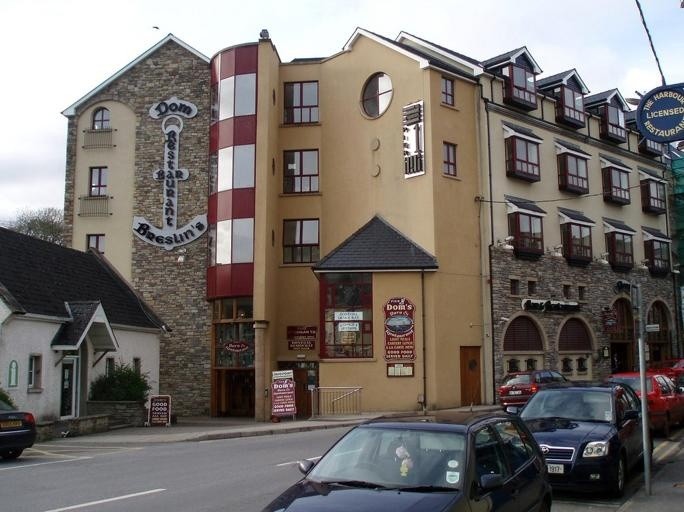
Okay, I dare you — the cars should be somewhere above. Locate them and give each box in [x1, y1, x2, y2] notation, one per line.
[0, 388, 35, 465]
[645, 358, 684, 387]
[518, 379, 655, 497]
[267, 412, 551, 510]
[497, 368, 567, 409]
[605, 370, 684, 436]
